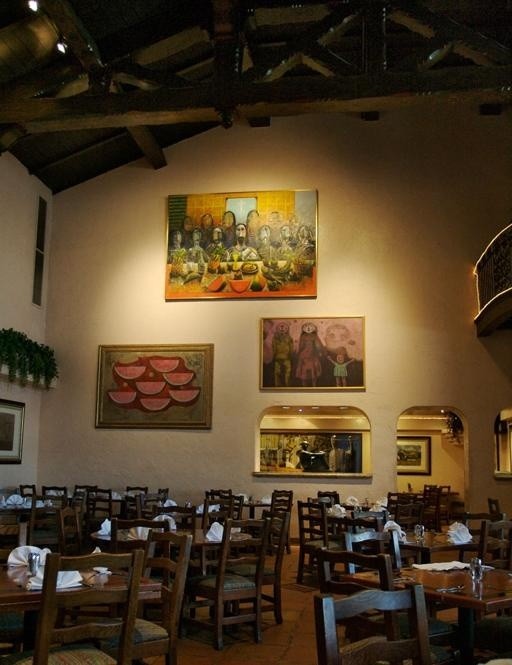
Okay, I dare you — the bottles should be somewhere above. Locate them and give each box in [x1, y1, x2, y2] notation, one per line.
[198, 253, 205, 275]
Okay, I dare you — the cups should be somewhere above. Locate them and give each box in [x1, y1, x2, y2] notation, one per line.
[297, 450, 325, 471]
[415, 525, 424, 540]
[470, 558, 484, 582]
[28, 552, 40, 575]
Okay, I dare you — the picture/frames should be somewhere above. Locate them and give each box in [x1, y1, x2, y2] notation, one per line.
[398, 434, 430, 475]
[166, 189, 319, 300]
[1, 399, 24, 464]
[259, 317, 366, 391]
[261, 429, 371, 472]
[97, 345, 213, 430]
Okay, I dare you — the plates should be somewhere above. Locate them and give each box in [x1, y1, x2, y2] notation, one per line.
[186, 256, 195, 273]
[241, 263, 258, 274]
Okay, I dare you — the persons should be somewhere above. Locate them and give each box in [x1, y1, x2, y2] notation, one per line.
[328, 433, 355, 473]
[295, 441, 328, 472]
